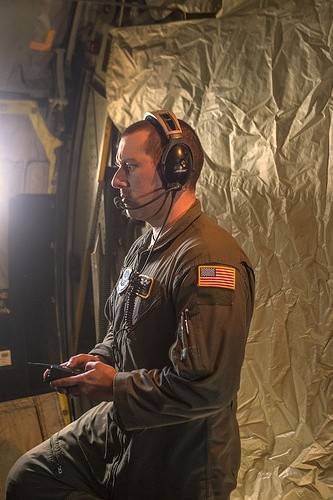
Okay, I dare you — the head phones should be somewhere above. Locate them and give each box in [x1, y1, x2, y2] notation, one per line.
[142, 109, 196, 191]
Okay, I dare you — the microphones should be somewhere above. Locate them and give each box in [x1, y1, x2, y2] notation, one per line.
[113, 189, 173, 211]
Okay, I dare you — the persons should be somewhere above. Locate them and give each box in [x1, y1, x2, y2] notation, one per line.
[2, 110, 256, 500]
